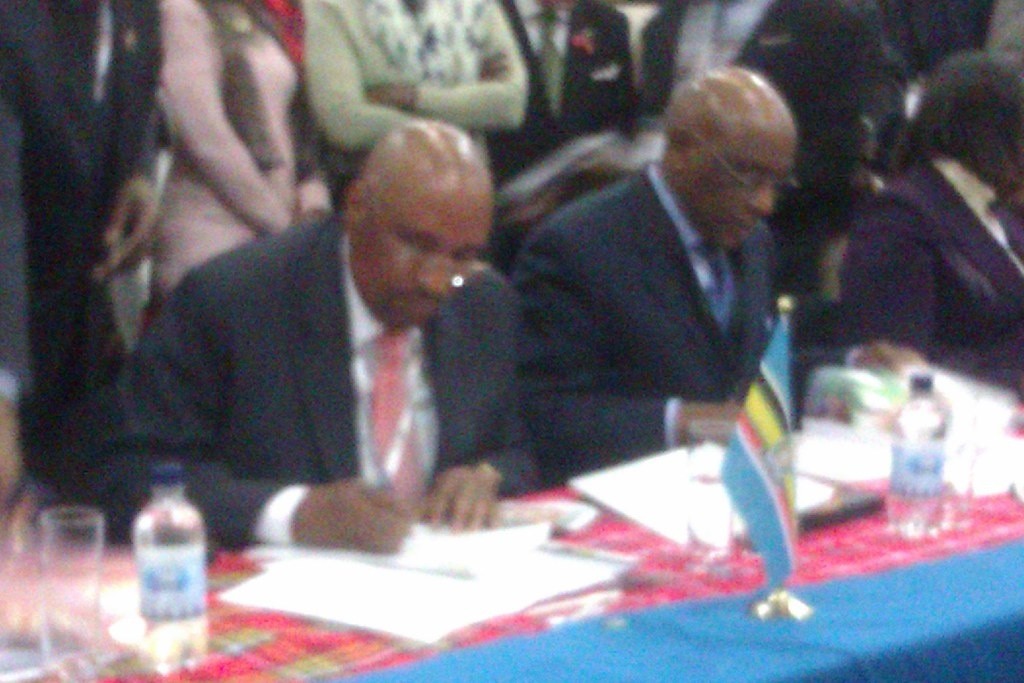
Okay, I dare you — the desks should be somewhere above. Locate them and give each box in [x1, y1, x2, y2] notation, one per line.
[0, 401, 1024, 683]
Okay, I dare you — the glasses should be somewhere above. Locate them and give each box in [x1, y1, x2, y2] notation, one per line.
[691, 133, 799, 203]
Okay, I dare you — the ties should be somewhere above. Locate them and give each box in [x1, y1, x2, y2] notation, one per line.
[695, 241, 736, 341]
[533, 8, 566, 120]
[991, 200, 1024, 266]
[365, 329, 427, 512]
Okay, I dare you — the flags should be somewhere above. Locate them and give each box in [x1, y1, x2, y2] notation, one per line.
[717, 314, 797, 589]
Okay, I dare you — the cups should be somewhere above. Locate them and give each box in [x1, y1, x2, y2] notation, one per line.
[36, 506, 106, 670]
[686, 421, 740, 563]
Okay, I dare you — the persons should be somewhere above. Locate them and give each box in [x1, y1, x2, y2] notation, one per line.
[838, 50, 1024, 406]
[509, 67, 926, 493]
[0, 0, 1023, 505]
[59, 120, 566, 553]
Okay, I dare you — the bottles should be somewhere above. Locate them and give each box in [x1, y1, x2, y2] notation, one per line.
[888, 374, 950, 542]
[128, 462, 210, 678]
